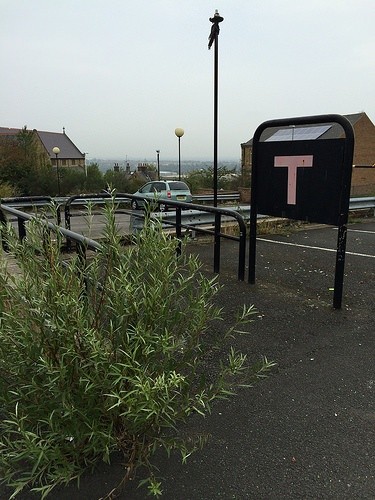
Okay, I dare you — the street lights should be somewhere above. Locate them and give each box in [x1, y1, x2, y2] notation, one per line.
[175, 128, 185, 180]
[52, 147, 60, 196]
[156, 149, 160, 180]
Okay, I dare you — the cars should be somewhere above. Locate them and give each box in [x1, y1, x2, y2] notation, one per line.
[130, 179, 192, 212]
